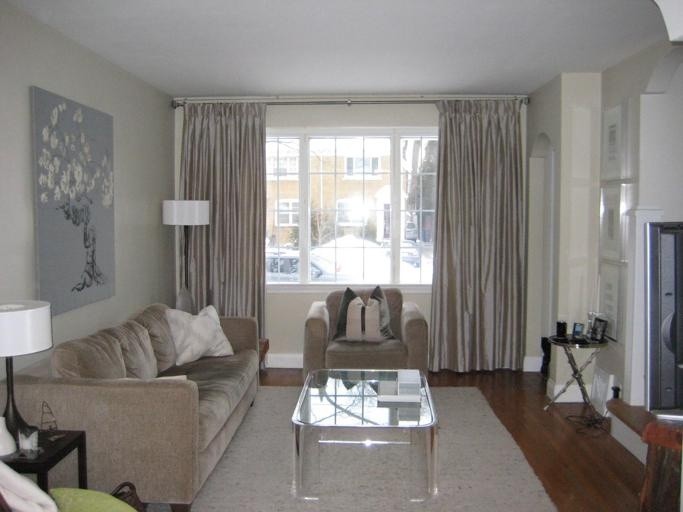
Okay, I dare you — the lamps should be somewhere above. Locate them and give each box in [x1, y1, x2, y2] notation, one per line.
[160, 201, 213, 290]
[0, 299, 52, 455]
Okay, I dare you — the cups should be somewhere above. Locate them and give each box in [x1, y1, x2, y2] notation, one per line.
[556, 322, 566, 339]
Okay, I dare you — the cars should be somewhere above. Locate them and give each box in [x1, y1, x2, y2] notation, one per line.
[380, 221, 420, 267]
[265, 247, 350, 282]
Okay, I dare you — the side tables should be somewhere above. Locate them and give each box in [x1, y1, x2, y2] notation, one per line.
[6, 429, 87, 501]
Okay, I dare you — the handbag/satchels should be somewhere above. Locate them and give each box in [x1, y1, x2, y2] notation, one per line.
[111, 482, 145, 512]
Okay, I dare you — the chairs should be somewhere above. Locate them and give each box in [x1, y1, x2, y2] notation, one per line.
[301, 286, 429, 391]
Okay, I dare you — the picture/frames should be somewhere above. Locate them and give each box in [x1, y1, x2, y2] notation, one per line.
[30, 85, 115, 317]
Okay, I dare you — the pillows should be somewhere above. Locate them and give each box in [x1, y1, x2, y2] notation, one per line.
[331, 283, 395, 344]
[47, 299, 234, 388]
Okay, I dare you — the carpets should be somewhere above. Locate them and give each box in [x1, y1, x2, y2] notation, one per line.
[139, 385, 558, 511]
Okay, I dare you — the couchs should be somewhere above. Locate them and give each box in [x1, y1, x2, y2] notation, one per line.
[0, 315, 263, 511]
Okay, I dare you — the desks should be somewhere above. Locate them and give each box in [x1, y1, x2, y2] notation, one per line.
[543, 334, 609, 414]
